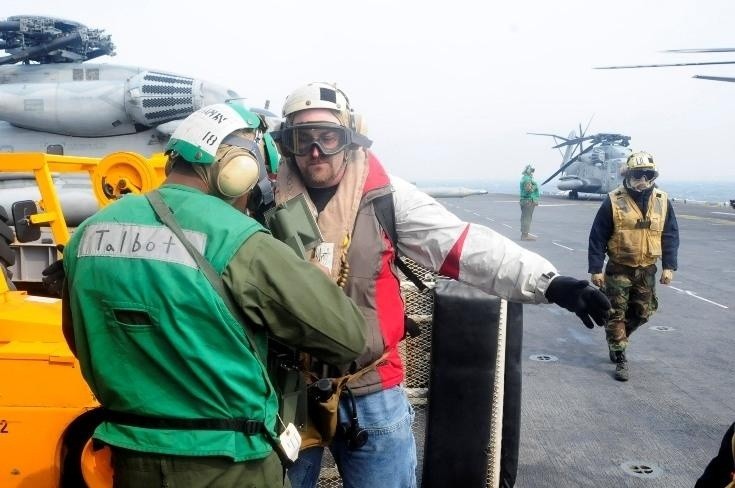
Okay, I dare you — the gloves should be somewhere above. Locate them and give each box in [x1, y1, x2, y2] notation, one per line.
[660, 270, 673, 284]
[545, 274, 610, 328]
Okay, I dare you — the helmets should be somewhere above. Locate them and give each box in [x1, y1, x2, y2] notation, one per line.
[282, 83, 355, 130]
[165, 102, 281, 199]
[621, 152, 657, 174]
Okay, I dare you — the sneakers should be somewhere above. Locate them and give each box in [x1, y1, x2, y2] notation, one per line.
[610, 349, 631, 381]
[521, 233, 538, 241]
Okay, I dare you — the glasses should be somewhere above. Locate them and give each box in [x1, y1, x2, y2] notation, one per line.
[280, 121, 353, 156]
[630, 170, 655, 179]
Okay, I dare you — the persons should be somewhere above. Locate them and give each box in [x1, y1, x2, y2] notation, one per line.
[518, 164, 541, 241]
[587, 150, 680, 383]
[38, 80, 611, 487]
[58, 101, 369, 487]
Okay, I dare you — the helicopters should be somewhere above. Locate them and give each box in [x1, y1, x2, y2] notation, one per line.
[595, 42, 735, 91]
[0, 6, 489, 289]
[526, 111, 635, 196]
